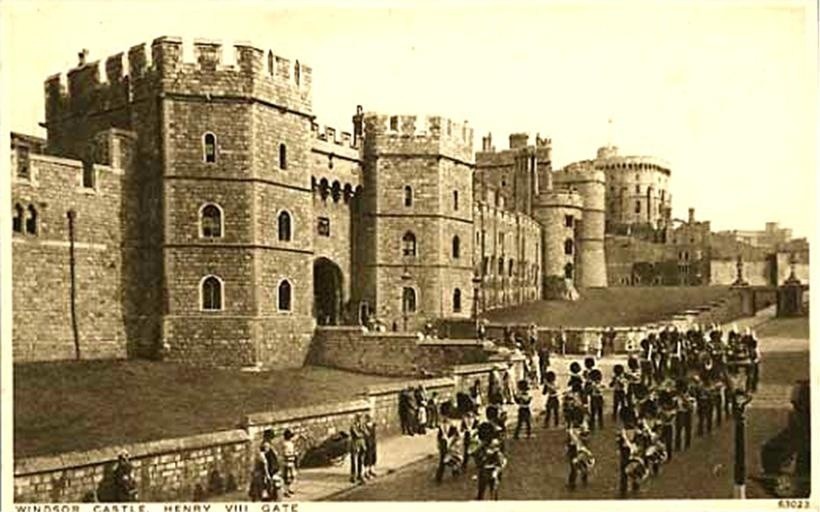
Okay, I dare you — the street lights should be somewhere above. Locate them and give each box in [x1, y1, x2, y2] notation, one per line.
[472, 269, 481, 340]
[722, 358, 752, 499]
[402, 266, 412, 332]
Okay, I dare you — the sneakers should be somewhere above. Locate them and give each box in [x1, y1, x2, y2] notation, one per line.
[348, 468, 378, 487]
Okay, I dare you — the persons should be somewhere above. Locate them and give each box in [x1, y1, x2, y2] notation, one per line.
[248, 440, 275, 501]
[351, 104, 365, 145]
[347, 411, 368, 485]
[359, 413, 377, 481]
[116, 449, 140, 501]
[278, 427, 299, 498]
[395, 311, 764, 498]
[259, 429, 280, 501]
